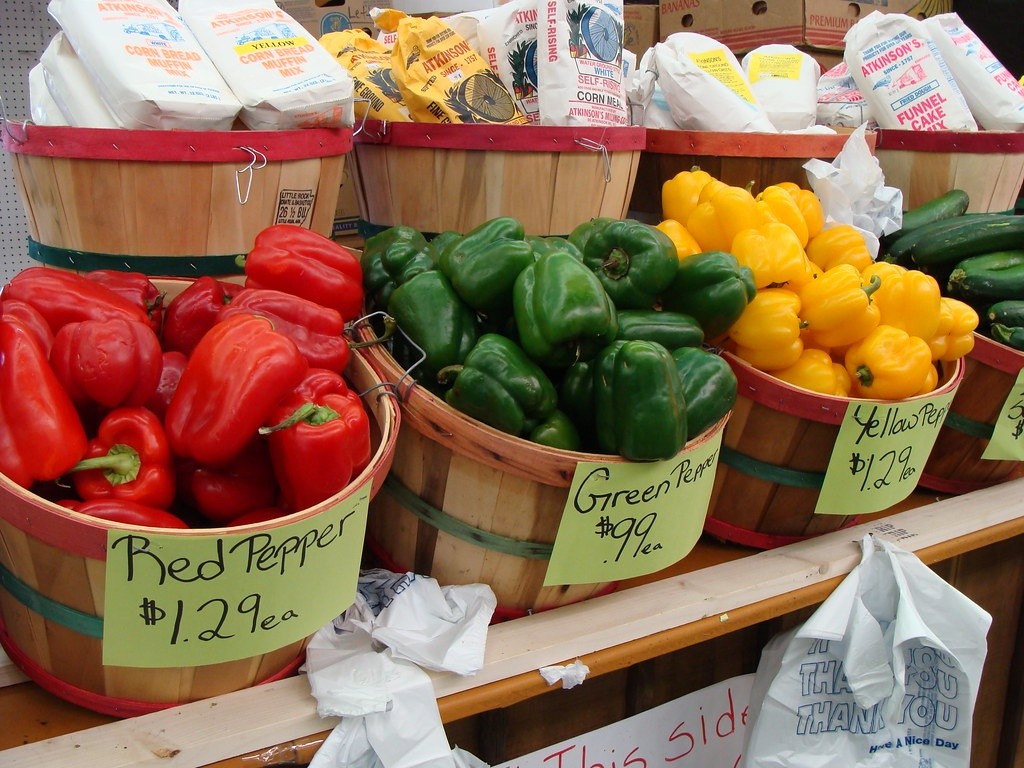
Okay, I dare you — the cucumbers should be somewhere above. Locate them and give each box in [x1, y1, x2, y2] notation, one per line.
[878, 190, 1024, 356]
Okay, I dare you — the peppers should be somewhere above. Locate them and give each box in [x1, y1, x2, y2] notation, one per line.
[0, 170, 979, 528]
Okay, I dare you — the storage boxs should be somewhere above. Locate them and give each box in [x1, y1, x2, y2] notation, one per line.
[278, 0, 962, 250]
